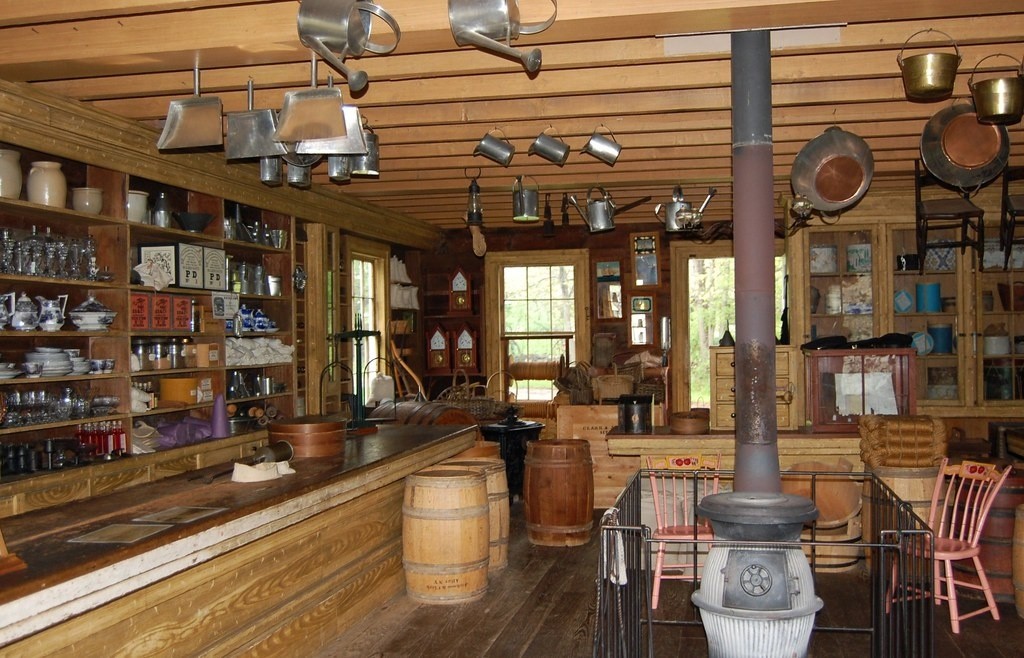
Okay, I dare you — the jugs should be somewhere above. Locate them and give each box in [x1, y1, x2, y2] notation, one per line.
[237, 260, 265, 295]
[448, 0, 557, 73]
[0, 291, 69, 331]
[296, 0, 402, 91]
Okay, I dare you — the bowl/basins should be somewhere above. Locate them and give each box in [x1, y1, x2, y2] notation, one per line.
[172, 212, 218, 232]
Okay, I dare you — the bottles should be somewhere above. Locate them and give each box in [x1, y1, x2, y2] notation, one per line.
[0, 223, 110, 280]
[152, 192, 171, 228]
[132, 380, 157, 413]
[77, 420, 127, 461]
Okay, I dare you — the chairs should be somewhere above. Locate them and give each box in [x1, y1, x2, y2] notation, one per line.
[915, 158, 985, 275]
[882, 457, 1012, 634]
[644, 450, 723, 610]
[997, 159, 1024, 272]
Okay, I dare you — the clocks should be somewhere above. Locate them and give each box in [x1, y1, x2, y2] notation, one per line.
[423, 265, 479, 375]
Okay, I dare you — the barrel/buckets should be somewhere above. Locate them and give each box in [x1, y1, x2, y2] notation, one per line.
[897, 28, 1024, 124]
[524, 439, 594, 546]
[370, 401, 510, 605]
[862, 464, 1024, 619]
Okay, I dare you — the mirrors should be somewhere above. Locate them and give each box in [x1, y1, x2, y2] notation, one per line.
[591, 230, 661, 351]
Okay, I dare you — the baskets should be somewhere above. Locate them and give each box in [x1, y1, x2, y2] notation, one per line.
[552, 352, 667, 405]
[432, 369, 524, 421]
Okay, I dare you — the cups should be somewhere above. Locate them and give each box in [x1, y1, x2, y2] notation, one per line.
[0, 390, 53, 430]
[471, 126, 622, 170]
[88, 358, 115, 375]
[22, 361, 43, 378]
[132, 387, 149, 413]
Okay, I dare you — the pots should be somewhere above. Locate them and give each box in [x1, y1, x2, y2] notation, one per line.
[790, 127, 875, 220]
[922, 97, 1010, 195]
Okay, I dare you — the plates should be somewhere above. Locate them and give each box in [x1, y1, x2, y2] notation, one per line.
[0, 346, 90, 380]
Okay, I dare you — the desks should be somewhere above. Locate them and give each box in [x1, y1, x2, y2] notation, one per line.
[606, 425, 863, 486]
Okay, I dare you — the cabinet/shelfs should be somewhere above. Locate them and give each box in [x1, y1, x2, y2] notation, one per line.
[295, 217, 353, 419]
[0, 139, 295, 520]
[783, 191, 1024, 435]
[707, 343, 801, 433]
